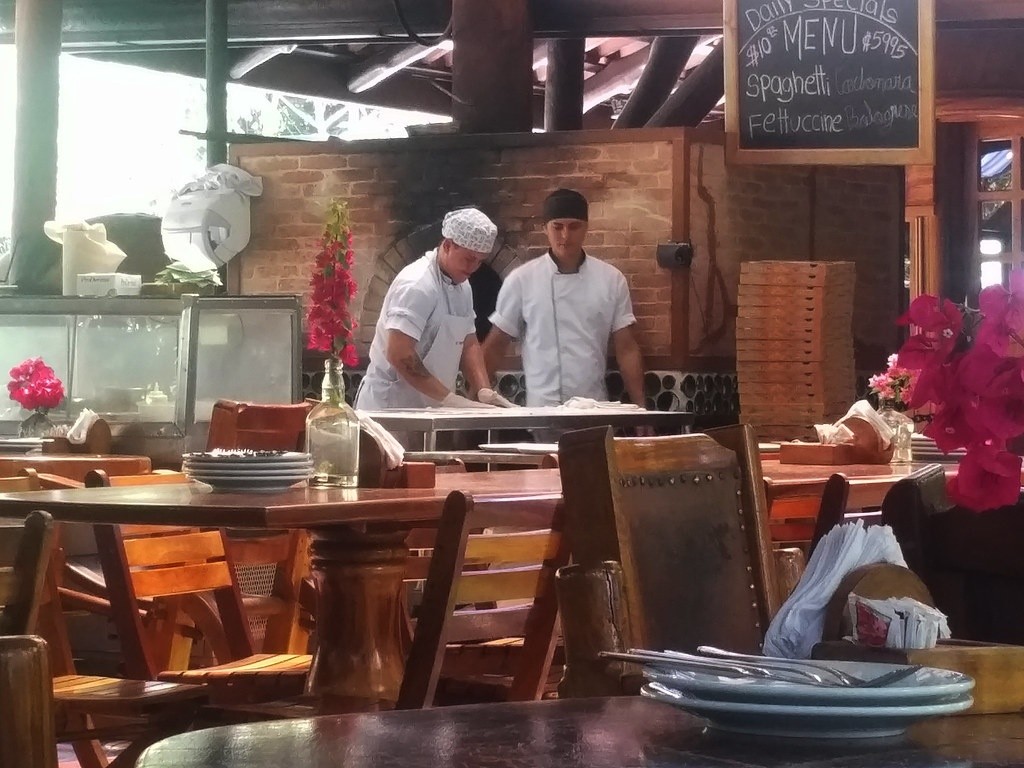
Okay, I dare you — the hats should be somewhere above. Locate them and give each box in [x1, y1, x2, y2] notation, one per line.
[542, 188, 588, 224]
[442, 207, 498, 253]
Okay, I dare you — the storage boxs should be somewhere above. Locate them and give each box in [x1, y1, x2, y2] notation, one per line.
[736, 259, 858, 442]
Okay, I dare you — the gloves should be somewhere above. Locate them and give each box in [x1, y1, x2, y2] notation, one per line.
[441, 391, 497, 408]
[478, 388, 521, 408]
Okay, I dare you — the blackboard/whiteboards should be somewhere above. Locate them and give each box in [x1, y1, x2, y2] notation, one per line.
[722, 0, 931, 163]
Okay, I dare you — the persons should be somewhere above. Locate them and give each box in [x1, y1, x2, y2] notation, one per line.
[352, 208, 520, 452]
[468, 188, 654, 443]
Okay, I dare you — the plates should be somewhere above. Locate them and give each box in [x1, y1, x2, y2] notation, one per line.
[911, 432, 968, 457]
[640, 661, 976, 737]
[183, 450, 315, 493]
[0, 437, 54, 451]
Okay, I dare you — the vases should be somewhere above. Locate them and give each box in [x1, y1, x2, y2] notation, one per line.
[876, 399, 915, 463]
[305, 359, 360, 490]
[19, 411, 56, 439]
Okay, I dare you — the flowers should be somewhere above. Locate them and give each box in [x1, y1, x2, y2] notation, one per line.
[894, 268, 1024, 513]
[307, 194, 360, 368]
[6, 358, 64, 416]
[868, 353, 921, 412]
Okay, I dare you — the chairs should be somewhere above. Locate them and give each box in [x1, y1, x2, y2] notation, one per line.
[0, 395, 1024, 768]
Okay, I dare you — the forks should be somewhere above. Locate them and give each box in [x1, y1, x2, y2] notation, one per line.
[599, 644, 922, 687]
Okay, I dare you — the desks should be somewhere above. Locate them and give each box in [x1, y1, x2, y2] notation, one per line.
[0, 463, 1024, 704]
[353, 406, 694, 471]
[135, 695, 1024, 768]
[0, 453, 152, 483]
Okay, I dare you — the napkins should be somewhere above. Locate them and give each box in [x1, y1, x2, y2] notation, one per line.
[762, 518, 908, 660]
[833, 400, 897, 451]
[354, 409, 405, 469]
[66, 408, 100, 445]
